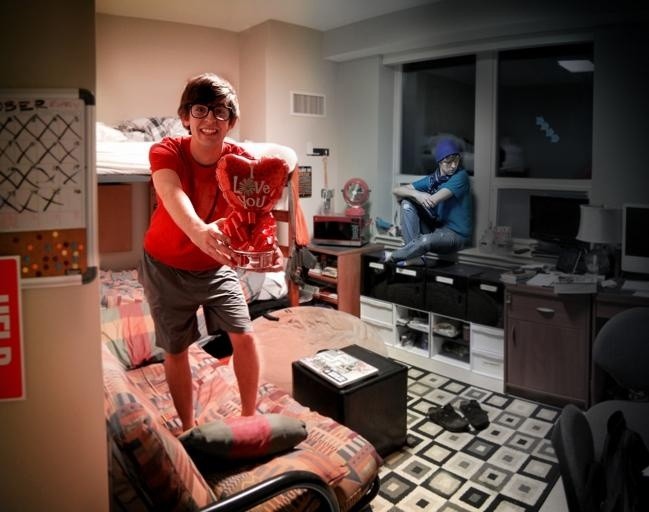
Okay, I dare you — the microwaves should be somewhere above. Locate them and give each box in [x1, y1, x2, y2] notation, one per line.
[312, 212, 371, 248]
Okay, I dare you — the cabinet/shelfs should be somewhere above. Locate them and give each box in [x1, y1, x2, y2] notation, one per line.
[355, 246, 504, 395]
[501, 275, 594, 414]
[295, 240, 387, 317]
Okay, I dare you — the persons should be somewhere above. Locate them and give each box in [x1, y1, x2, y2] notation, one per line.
[139, 69, 259, 432]
[375, 141, 473, 274]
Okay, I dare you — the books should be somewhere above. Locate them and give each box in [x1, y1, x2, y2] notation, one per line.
[499, 270, 598, 295]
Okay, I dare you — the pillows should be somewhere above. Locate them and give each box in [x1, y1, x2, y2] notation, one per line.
[174, 411, 307, 468]
[97, 297, 168, 366]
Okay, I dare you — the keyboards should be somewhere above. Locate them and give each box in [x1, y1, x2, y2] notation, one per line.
[620, 278, 649, 292]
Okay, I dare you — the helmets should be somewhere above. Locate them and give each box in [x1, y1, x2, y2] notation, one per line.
[433, 139, 462, 162]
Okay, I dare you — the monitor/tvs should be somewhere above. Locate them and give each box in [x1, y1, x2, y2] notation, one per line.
[528, 194, 588, 242]
[622, 203, 649, 273]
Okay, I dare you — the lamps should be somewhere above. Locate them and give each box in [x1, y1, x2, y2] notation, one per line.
[573, 203, 610, 275]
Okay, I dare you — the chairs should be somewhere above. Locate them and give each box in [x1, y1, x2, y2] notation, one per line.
[579, 305, 648, 465]
[548, 403, 648, 511]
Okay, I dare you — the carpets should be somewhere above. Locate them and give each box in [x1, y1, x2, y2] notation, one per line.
[370, 353, 574, 512]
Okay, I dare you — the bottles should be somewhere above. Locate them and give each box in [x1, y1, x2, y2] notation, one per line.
[400, 336, 407, 346]
[479, 222, 495, 254]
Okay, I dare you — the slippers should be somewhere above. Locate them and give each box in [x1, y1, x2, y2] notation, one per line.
[429, 398, 491, 432]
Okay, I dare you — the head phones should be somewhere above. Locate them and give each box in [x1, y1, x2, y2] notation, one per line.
[430, 169, 451, 186]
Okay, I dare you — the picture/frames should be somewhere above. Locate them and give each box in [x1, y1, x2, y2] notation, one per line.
[1, 86, 99, 293]
[557, 244, 584, 274]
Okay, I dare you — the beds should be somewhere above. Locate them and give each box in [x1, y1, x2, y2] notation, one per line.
[96, 121, 304, 372]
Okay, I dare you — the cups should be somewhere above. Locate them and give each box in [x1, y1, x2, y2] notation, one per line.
[463, 326, 470, 343]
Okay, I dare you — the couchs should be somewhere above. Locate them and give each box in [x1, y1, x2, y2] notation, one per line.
[98, 331, 385, 510]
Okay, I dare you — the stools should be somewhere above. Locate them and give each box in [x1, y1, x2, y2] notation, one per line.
[229, 303, 389, 396]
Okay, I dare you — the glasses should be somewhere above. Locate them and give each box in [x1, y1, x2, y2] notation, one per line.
[187, 101, 232, 123]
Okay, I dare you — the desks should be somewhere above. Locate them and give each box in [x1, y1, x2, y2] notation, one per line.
[591, 283, 649, 413]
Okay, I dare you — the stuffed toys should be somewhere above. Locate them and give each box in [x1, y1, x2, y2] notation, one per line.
[215, 154, 289, 266]
